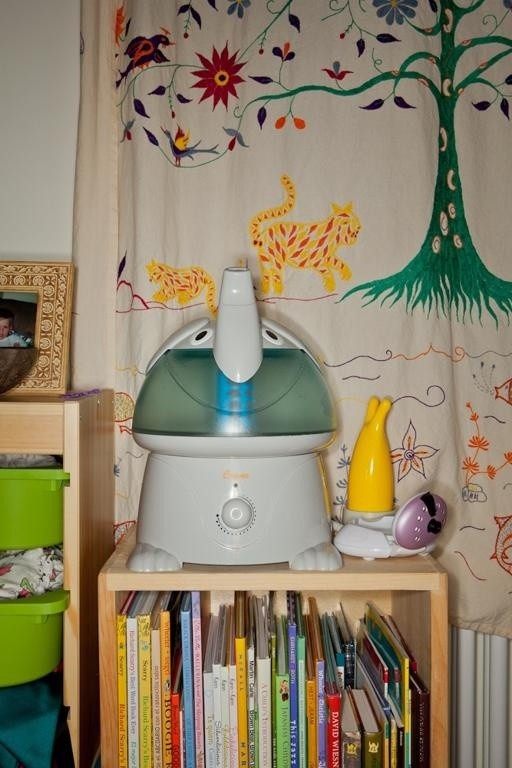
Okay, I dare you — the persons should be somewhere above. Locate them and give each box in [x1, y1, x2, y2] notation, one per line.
[0, 307, 28, 347]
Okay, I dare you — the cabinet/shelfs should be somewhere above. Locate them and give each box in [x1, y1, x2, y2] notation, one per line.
[0, 387, 116, 767]
[96, 572, 449, 768]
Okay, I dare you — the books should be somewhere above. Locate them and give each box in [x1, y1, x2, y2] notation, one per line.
[113, 589, 434, 768]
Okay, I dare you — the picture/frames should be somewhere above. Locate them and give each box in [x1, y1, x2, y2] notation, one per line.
[0, 260, 74, 397]
